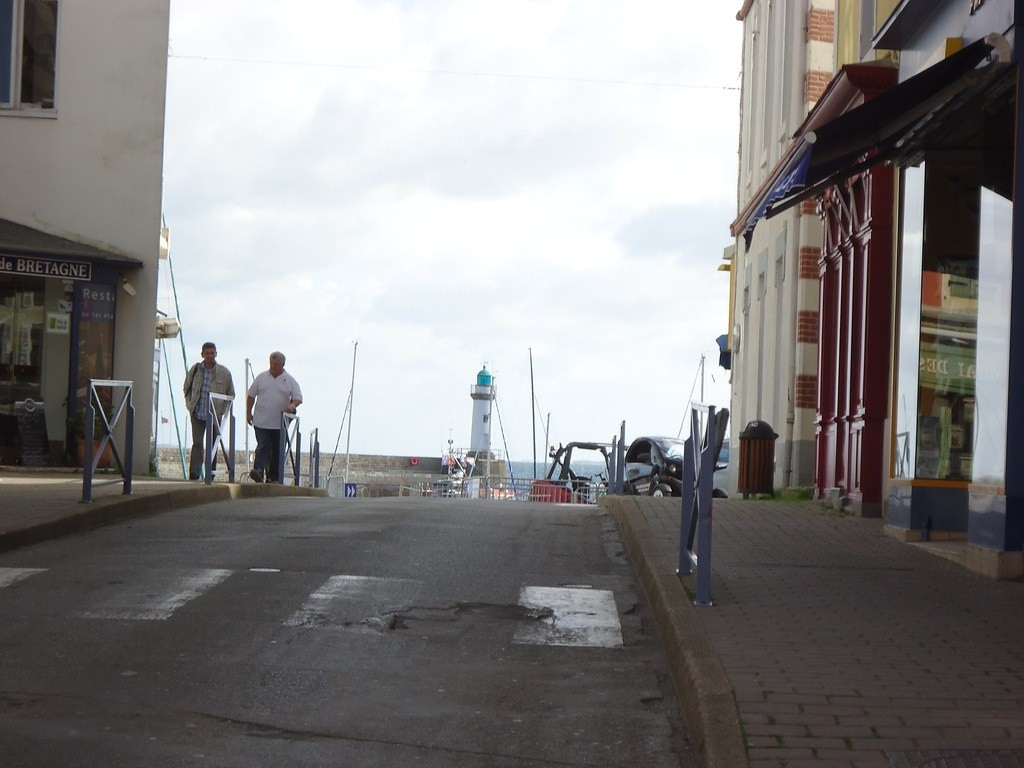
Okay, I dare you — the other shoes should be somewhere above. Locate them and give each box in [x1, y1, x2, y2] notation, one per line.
[190, 472, 199, 480]
[250, 467, 263, 483]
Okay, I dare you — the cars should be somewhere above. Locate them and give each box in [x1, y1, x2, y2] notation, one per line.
[713, 438, 729, 499]
[623, 436, 686, 497]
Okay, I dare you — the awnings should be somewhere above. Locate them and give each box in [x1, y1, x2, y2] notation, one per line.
[746, 32, 1011, 232]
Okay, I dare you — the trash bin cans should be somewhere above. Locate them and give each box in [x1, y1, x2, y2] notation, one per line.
[736, 422, 779, 500]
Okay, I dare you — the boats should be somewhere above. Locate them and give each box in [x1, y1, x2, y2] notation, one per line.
[428, 347, 629, 502]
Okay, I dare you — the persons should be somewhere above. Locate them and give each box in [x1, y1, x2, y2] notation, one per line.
[183, 343, 235, 478]
[245, 352, 302, 483]
